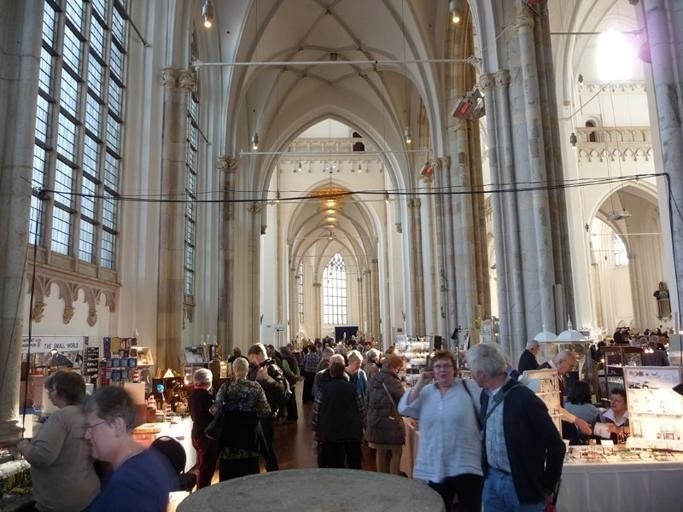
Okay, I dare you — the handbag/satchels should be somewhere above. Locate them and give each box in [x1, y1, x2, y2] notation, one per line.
[363, 410, 406, 445]
[203, 380, 233, 442]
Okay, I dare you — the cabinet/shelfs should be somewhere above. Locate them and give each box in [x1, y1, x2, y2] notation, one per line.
[599, 346, 664, 401]
[84, 347, 99, 375]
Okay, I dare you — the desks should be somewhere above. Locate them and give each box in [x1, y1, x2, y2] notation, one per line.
[555, 444, 683, 512]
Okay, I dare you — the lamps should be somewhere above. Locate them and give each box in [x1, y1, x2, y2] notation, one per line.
[600, 86, 633, 221]
[534, 323, 556, 343]
[552, 315, 590, 345]
[578, 74, 583, 85]
[451, 86, 482, 120]
[250, 0, 259, 150]
[201, 0, 214, 28]
[570, 133, 577, 148]
[403, 0, 413, 144]
[449, 0, 461, 23]
[421, 155, 440, 178]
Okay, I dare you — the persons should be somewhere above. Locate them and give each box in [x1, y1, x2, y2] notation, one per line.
[466, 342, 566, 512]
[397, 351, 486, 512]
[518, 340, 630, 446]
[15, 369, 180, 512]
[188, 336, 405, 491]
[641, 335, 669, 366]
